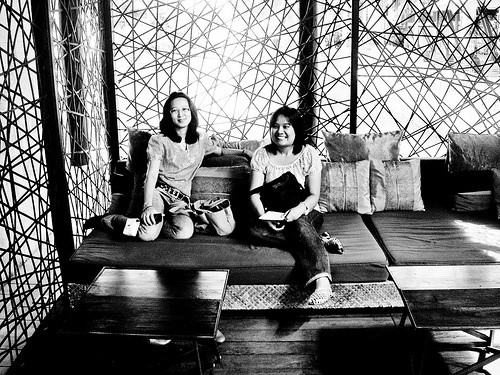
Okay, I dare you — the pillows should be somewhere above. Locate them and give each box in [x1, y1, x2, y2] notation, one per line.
[125, 128, 500, 222]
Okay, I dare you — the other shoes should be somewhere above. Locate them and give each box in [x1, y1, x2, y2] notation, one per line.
[321, 231, 344, 254]
[307, 289, 335, 304]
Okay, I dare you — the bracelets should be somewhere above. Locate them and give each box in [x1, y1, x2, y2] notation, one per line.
[300, 202, 309, 215]
[242, 149, 245, 154]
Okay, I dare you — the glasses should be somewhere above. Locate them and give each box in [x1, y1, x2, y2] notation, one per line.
[169, 107, 190, 114]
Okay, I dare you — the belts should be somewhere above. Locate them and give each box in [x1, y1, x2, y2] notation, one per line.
[158, 182, 189, 202]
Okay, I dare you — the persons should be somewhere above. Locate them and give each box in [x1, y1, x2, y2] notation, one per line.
[249, 106, 332, 305]
[83, 91, 251, 242]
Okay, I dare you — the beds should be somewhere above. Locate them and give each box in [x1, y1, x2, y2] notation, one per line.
[362, 160, 500, 314]
[67, 161, 389, 319]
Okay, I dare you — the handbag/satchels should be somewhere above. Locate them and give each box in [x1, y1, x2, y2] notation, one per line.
[249, 170, 307, 211]
[194, 196, 235, 235]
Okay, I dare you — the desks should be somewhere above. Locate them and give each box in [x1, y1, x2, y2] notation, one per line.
[384, 262, 500, 375]
[56, 265, 230, 375]
